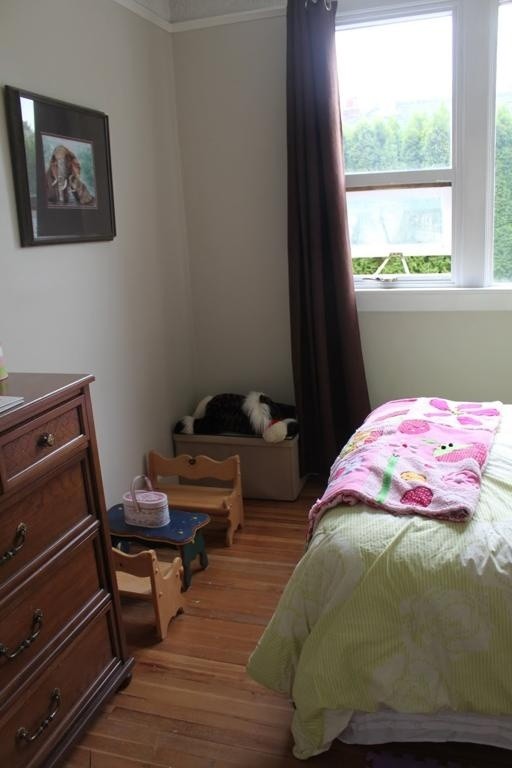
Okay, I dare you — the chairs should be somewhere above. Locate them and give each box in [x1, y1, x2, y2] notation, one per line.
[143, 448, 246, 548]
[111, 548, 188, 642]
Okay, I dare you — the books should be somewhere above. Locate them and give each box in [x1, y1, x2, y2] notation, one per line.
[1, 395, 24, 416]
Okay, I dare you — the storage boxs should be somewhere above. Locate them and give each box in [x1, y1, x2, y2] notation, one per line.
[172, 432, 311, 502]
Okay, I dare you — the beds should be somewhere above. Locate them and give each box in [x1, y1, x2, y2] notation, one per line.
[246, 394, 512, 760]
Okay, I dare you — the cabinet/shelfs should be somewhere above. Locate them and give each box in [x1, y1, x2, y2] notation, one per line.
[0, 371, 137, 767]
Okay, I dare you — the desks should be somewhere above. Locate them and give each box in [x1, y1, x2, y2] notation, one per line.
[107, 501, 210, 594]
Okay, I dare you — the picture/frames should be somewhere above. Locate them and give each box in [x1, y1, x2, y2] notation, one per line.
[2, 83, 117, 248]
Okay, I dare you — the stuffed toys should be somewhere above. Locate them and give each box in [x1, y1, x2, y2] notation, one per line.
[174, 391, 298, 444]
